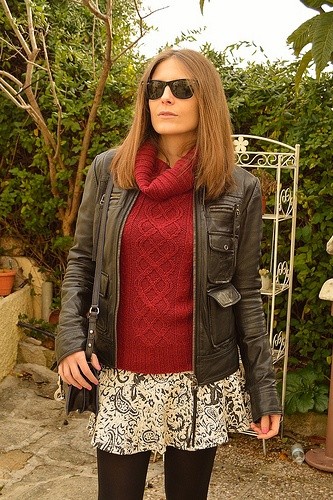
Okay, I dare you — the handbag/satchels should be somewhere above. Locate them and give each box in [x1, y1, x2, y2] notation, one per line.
[66, 358, 100, 418]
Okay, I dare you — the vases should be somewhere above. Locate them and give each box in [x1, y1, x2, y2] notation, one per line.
[0, 269, 16, 296]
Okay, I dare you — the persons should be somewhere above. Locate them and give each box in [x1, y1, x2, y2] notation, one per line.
[56, 49, 283, 500]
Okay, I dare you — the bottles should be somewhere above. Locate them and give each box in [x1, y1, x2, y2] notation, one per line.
[292, 444, 305, 466]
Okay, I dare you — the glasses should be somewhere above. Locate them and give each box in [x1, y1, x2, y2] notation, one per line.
[146, 79, 198, 100]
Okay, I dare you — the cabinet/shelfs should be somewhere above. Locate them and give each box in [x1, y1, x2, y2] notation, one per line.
[232, 134, 300, 455]
